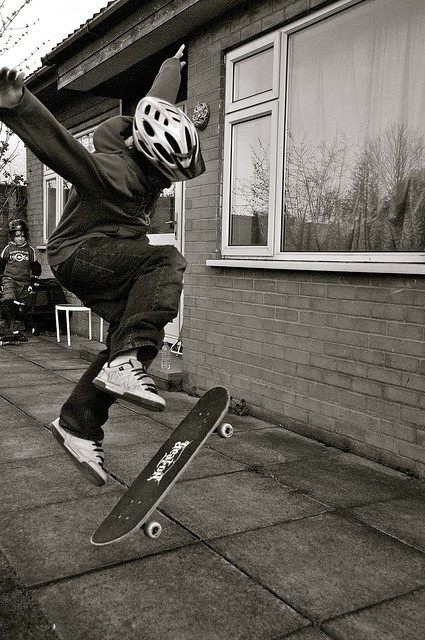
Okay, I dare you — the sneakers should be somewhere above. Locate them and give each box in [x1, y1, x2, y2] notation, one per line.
[48, 416, 108, 487]
[91, 357, 167, 413]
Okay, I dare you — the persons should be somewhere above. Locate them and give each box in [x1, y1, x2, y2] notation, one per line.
[1, 218, 42, 342]
[0, 44, 206, 491]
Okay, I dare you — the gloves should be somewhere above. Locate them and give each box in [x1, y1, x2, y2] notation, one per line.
[29, 278, 38, 291]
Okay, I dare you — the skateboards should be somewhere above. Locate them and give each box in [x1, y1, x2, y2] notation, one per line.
[91, 386, 233, 546]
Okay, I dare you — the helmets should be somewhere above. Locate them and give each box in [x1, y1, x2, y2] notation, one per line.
[132, 96, 206, 183]
[6, 219, 29, 232]
